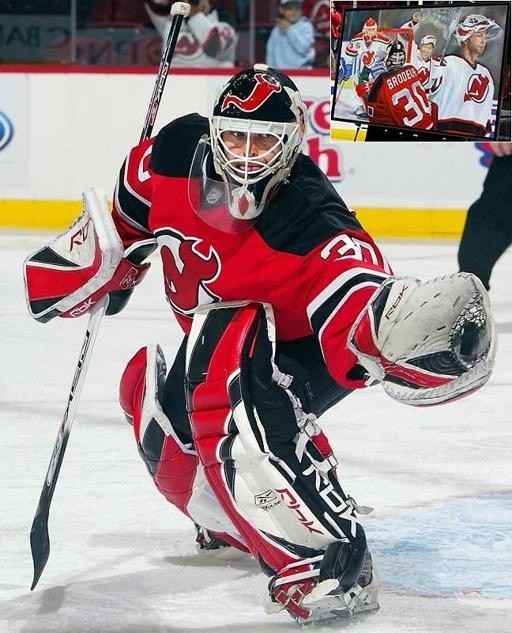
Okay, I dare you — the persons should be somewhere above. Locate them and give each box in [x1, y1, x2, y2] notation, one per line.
[22, 63, 495, 612]
[265, 0, 317, 69]
[331, 2, 504, 136]
[144, 1, 238, 68]
[460, 144, 512, 293]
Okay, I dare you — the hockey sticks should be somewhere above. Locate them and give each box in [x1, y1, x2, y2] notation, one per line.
[30, 2, 191, 593]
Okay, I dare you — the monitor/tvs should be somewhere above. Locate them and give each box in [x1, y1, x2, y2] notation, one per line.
[331, 1, 511, 141]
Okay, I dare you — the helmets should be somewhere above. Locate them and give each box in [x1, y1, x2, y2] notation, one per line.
[207, 64, 309, 222]
[384, 41, 408, 71]
[364, 19, 379, 35]
[422, 34, 438, 46]
[454, 15, 503, 45]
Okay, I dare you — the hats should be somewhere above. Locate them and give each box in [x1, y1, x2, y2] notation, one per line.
[278, 0, 303, 9]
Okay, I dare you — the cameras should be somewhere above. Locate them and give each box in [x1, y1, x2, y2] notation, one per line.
[188, 0, 200, 6]
